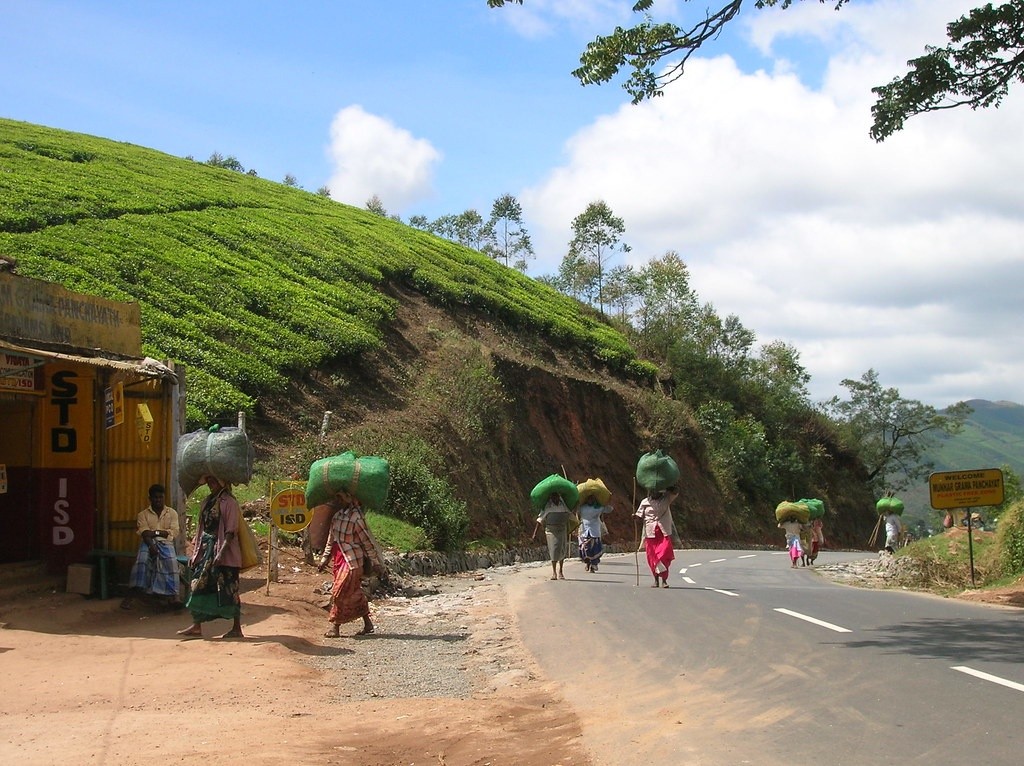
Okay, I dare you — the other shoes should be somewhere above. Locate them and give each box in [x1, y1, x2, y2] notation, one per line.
[120, 597, 140, 609]
[651, 581, 669, 588]
[175, 628, 204, 638]
[323, 629, 340, 638]
[355, 626, 374, 635]
[222, 628, 244, 638]
[585, 564, 590, 571]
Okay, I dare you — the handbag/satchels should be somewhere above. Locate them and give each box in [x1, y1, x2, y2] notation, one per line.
[800, 540, 806, 544]
[817, 531, 823, 544]
[363, 553, 373, 575]
[567, 512, 580, 535]
[235, 503, 263, 573]
[600, 520, 609, 535]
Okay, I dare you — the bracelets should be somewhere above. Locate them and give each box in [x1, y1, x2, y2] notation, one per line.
[155, 531, 160, 535]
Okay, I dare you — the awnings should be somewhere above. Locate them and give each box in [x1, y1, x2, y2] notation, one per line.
[0, 334, 172, 391]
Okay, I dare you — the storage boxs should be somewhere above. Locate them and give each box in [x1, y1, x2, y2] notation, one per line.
[65, 564, 96, 596]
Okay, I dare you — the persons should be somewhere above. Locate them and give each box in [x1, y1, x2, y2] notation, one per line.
[879, 510, 902, 552]
[120, 485, 179, 610]
[778, 515, 826, 568]
[318, 488, 381, 636]
[576, 495, 613, 573]
[631, 486, 680, 587]
[532, 492, 581, 580]
[175, 476, 242, 637]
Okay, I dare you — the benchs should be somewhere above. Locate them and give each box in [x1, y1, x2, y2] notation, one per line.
[87, 548, 190, 604]
